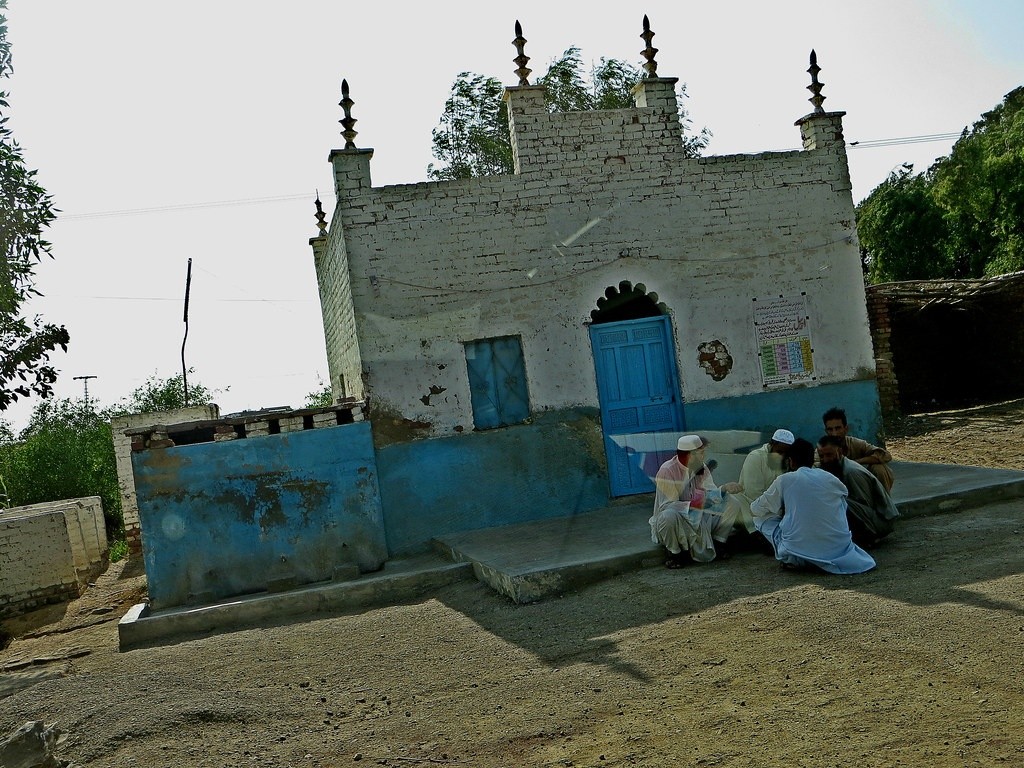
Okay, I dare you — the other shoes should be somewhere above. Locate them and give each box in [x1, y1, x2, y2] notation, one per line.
[665, 554, 681, 570]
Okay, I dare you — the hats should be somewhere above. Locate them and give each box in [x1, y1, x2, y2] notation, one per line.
[771, 429, 795, 445]
[677, 435, 703, 451]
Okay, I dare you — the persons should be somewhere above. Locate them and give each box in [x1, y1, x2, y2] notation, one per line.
[730, 429, 795, 557]
[653, 434, 741, 570]
[750, 437, 877, 575]
[814, 406, 894, 496]
[814, 435, 902, 550]
